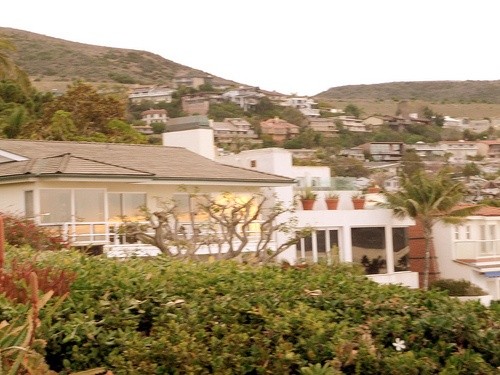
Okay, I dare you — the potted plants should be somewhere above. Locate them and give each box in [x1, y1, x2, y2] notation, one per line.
[324, 192, 339, 210]
[351, 190, 367, 211]
[297, 184, 318, 211]
